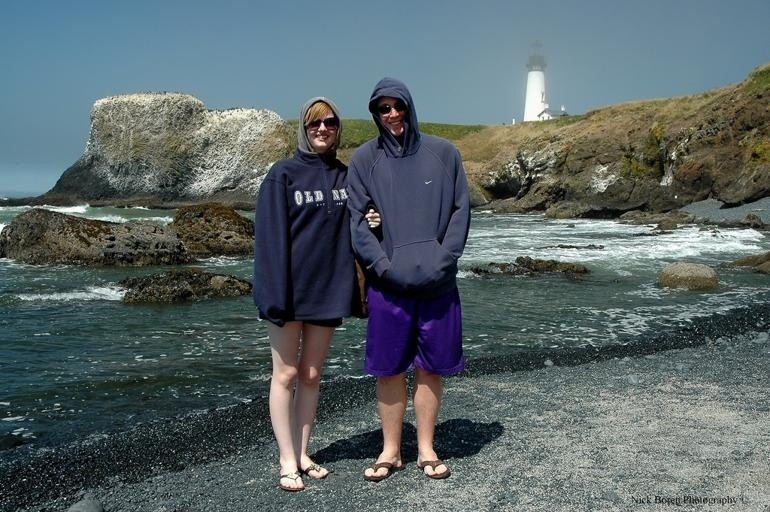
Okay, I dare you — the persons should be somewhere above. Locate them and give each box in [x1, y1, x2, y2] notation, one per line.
[252, 96, 380, 491]
[347, 77, 471, 481]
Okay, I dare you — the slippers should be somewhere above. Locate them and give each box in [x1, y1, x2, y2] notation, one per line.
[416, 456, 451, 479]
[363, 458, 406, 481]
[301, 461, 330, 480]
[279, 469, 305, 492]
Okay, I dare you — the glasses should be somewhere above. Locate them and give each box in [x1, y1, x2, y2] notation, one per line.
[376, 101, 406, 114]
[308, 118, 339, 131]
